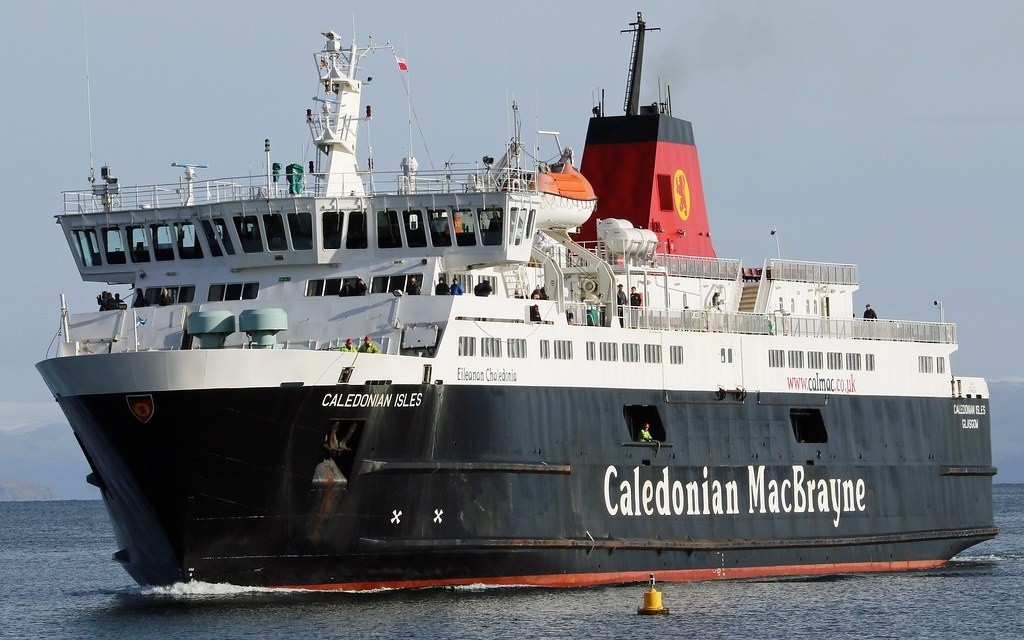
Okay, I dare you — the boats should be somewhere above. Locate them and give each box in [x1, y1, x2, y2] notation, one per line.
[495, 164, 597, 230]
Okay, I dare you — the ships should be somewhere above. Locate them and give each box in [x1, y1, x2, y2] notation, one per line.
[33, 11, 999, 591]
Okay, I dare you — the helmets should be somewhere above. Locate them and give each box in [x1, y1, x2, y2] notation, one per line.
[364, 336, 371, 340]
[346, 339, 352, 344]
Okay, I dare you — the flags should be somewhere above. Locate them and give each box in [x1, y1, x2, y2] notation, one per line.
[136, 316, 147, 326]
[396, 58, 408, 71]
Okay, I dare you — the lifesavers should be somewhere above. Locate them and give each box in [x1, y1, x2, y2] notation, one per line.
[583, 280, 596, 292]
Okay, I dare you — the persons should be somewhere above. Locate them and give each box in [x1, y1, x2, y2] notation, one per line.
[638, 423, 652, 442]
[435, 277, 463, 295]
[96, 291, 124, 312]
[864, 305, 878, 322]
[432, 212, 448, 246]
[617, 284, 627, 328]
[357, 336, 378, 353]
[521, 284, 546, 321]
[158, 288, 173, 305]
[133, 288, 145, 307]
[631, 286, 642, 309]
[475, 280, 492, 321]
[489, 212, 500, 229]
[339, 278, 367, 296]
[408, 278, 420, 295]
[340, 339, 357, 352]
